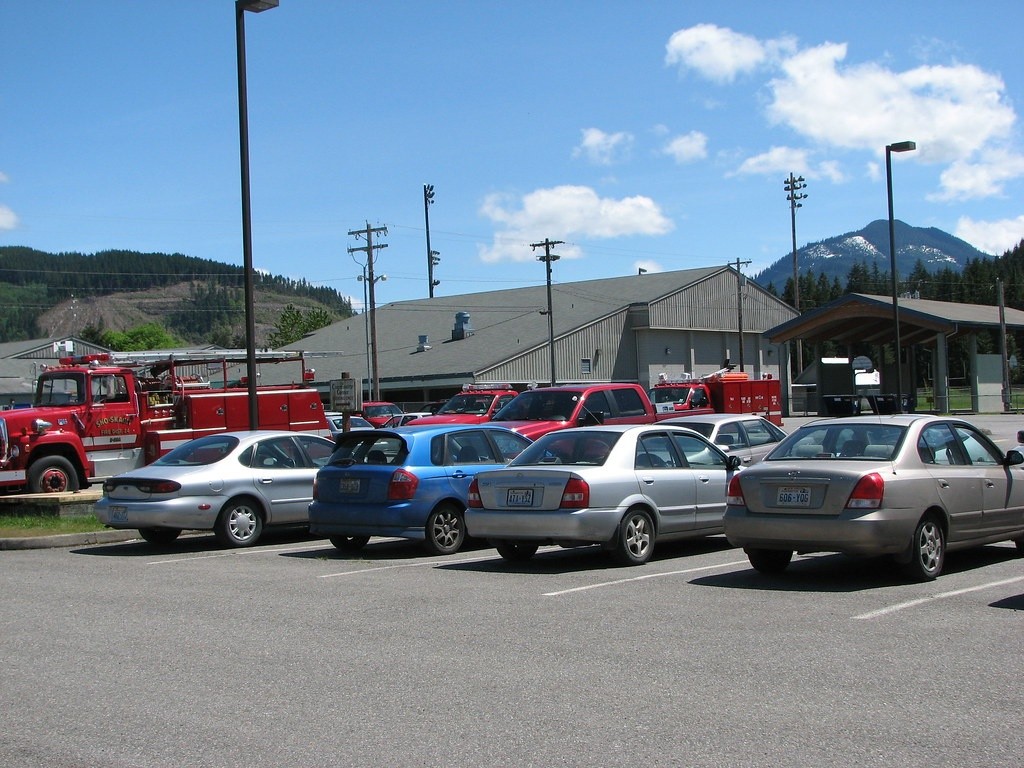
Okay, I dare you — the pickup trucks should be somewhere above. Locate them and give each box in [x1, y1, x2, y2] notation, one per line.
[480, 382, 715, 462]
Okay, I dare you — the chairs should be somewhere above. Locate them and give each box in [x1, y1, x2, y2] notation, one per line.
[466, 399, 508, 411]
[367, 450, 387, 464]
[801, 439, 938, 463]
[459, 444, 482, 462]
[639, 451, 668, 469]
[559, 399, 585, 419]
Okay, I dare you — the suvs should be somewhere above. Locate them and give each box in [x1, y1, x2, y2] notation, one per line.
[353, 402, 408, 430]
[405, 383, 520, 426]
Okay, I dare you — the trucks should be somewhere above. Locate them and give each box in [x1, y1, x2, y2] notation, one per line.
[645, 372, 784, 428]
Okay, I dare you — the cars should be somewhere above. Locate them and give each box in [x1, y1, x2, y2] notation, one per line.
[463, 424, 750, 568]
[637, 413, 816, 468]
[379, 412, 432, 429]
[324, 412, 389, 456]
[307, 424, 559, 555]
[93, 429, 369, 549]
[723, 414, 1024, 584]
[894, 411, 993, 463]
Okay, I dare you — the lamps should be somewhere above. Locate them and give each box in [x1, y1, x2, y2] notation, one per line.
[667, 348, 670, 354]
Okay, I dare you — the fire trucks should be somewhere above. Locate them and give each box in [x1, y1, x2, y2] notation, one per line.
[0, 347, 333, 512]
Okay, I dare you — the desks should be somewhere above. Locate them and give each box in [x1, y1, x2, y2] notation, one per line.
[822, 394, 904, 420]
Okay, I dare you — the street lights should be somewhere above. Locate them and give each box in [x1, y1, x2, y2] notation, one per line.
[235, 0, 280, 432]
[782, 170, 808, 377]
[423, 182, 440, 298]
[885, 141, 916, 414]
